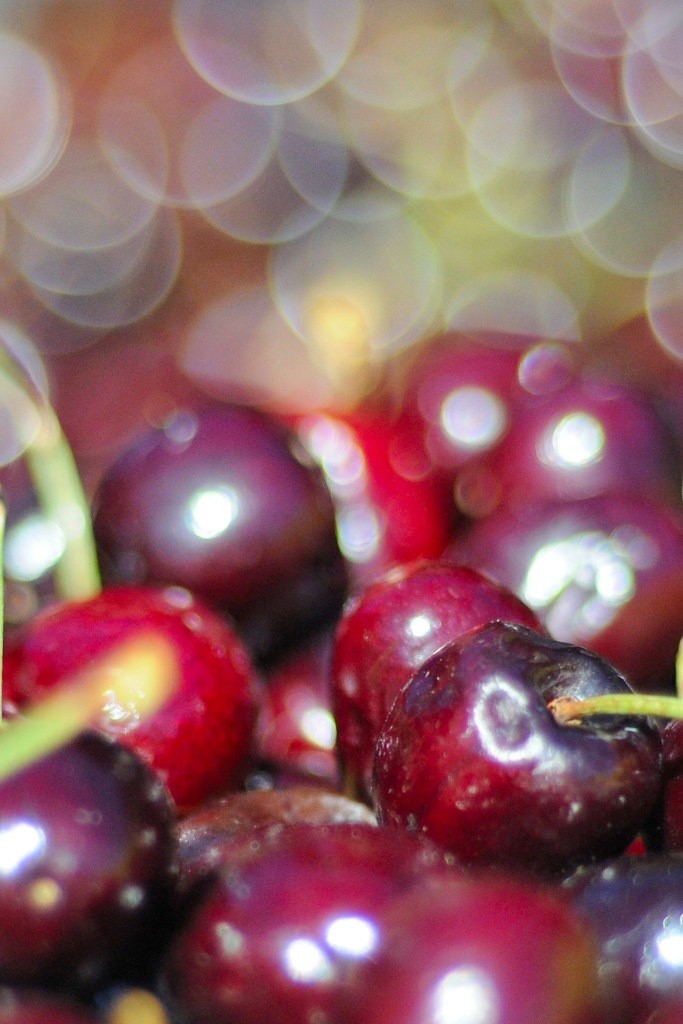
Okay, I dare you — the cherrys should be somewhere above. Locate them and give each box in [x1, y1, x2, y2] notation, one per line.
[0, 329, 683, 1024]
